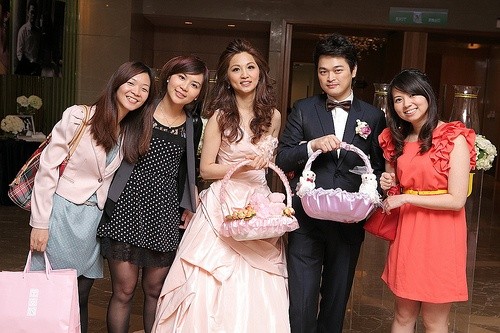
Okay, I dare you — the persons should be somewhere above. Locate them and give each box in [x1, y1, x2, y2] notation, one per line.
[150, 40, 291, 333]
[15, 0, 42, 70]
[275, 35, 387, 333]
[25, 61, 155, 333]
[380, 67, 476, 333]
[100, 56, 210, 333]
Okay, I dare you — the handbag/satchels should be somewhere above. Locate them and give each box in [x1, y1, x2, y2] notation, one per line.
[362, 138, 401, 242]
[0, 248, 81, 333]
[8, 105, 90, 211]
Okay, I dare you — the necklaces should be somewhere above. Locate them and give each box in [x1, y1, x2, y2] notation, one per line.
[161, 105, 182, 128]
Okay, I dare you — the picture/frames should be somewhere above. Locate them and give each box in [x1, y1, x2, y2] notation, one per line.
[16, 115, 36, 136]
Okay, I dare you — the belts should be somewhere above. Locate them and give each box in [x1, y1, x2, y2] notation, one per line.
[402, 190, 448, 195]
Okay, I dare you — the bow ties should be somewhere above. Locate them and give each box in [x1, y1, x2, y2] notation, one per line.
[326, 99, 351, 113]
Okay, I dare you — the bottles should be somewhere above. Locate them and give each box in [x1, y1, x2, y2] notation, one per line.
[371, 82, 401, 132]
[449, 84, 482, 135]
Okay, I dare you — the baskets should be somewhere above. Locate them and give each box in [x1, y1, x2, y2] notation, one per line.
[220, 159, 299, 241]
[296, 143, 383, 223]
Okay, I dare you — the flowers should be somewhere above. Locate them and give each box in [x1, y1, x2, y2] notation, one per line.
[355, 118, 371, 138]
[224, 202, 258, 220]
[475, 134, 497, 171]
[16, 95, 43, 114]
[1, 115, 24, 135]
